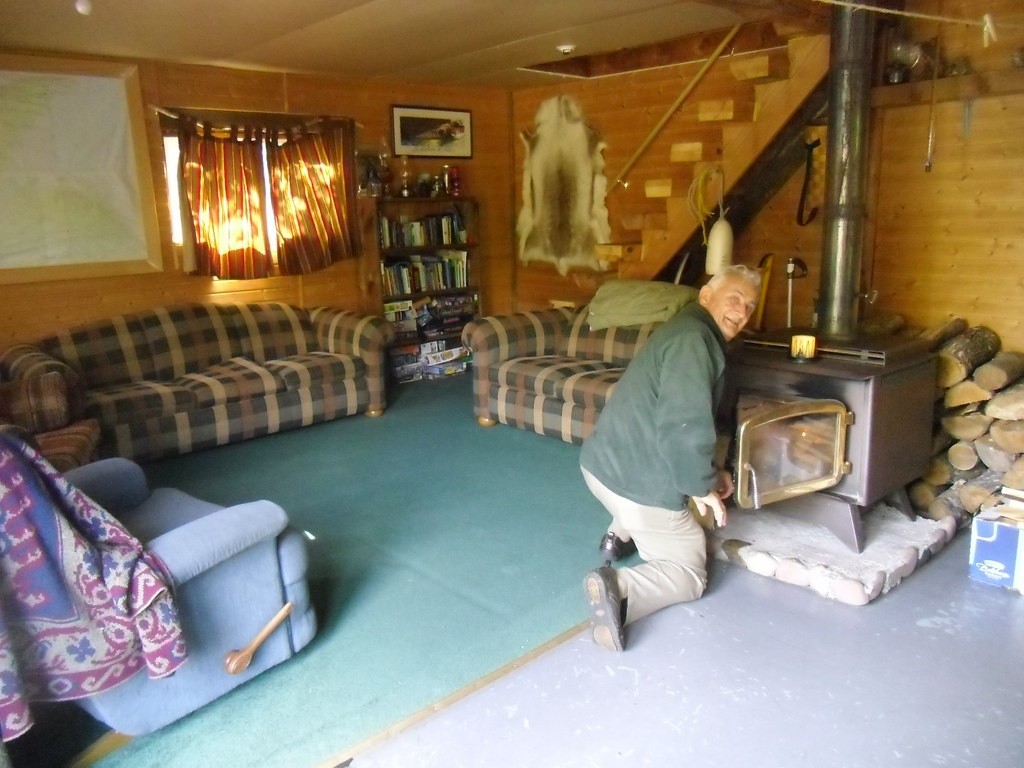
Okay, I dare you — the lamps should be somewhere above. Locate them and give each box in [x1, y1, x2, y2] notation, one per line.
[792, 334, 816, 365]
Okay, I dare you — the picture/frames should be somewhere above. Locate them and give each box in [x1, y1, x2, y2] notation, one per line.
[390, 104, 473, 159]
[0, 54, 164, 285]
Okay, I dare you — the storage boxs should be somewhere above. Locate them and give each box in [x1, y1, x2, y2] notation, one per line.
[969, 503, 1024, 594]
[797, 120, 827, 225]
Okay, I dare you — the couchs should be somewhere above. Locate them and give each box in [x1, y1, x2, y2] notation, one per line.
[31, 419, 99, 475]
[0, 302, 392, 467]
[462, 309, 749, 479]
[0, 427, 318, 737]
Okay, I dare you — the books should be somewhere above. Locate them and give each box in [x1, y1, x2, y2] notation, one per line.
[389, 339, 474, 385]
[377, 212, 471, 249]
[380, 249, 472, 296]
[383, 295, 477, 341]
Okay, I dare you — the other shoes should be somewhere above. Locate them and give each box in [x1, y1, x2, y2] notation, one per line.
[586, 532, 636, 651]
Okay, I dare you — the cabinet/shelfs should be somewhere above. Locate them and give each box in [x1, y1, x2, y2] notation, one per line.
[374, 195, 483, 388]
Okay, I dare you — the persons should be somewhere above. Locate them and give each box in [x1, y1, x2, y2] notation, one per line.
[578, 264, 764, 651]
